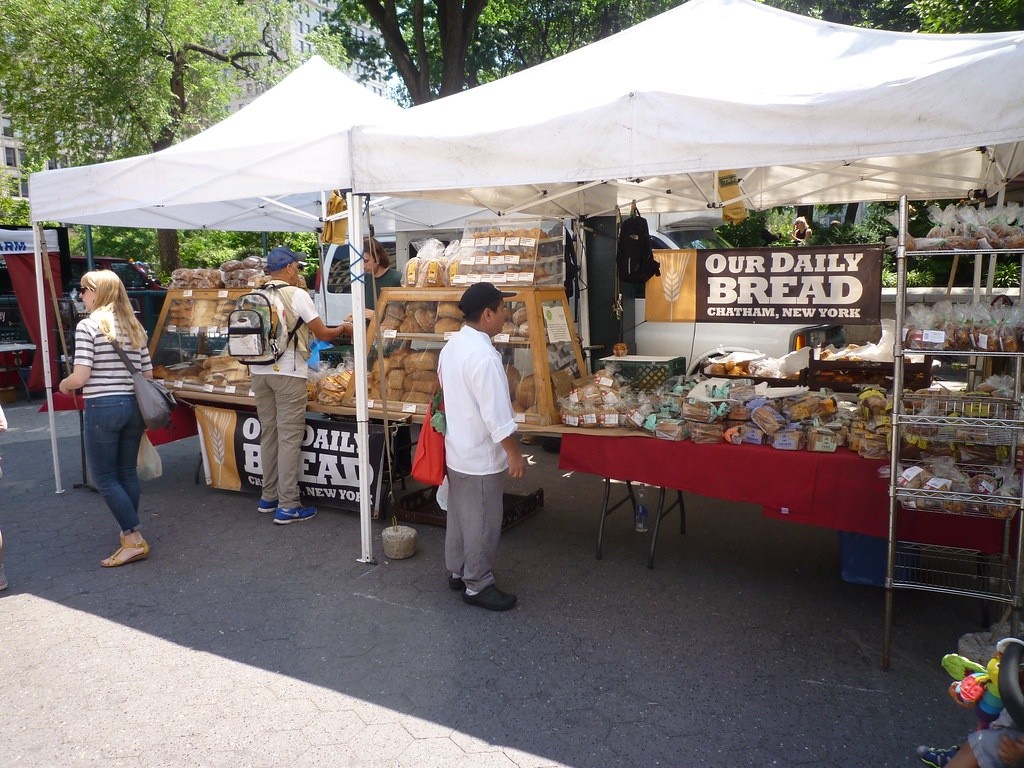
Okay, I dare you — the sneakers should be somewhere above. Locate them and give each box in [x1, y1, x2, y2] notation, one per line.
[273, 507, 318, 524]
[257, 499, 280, 513]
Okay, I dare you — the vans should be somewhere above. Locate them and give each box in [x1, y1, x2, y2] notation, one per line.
[308, 236, 397, 346]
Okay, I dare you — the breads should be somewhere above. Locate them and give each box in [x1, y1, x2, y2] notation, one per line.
[366, 227, 557, 417]
[151, 255, 354, 405]
[896, 221, 1024, 520]
[561, 343, 925, 458]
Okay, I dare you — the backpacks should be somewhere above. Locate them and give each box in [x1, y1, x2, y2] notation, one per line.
[616, 207, 661, 284]
[227, 282, 304, 375]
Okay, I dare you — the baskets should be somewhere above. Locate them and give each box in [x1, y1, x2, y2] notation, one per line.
[595, 356, 686, 397]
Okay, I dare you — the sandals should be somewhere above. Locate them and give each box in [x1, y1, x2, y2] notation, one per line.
[100, 538, 150, 567]
[917, 745, 960, 768]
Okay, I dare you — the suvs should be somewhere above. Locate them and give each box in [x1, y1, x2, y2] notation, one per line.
[0, 255, 168, 348]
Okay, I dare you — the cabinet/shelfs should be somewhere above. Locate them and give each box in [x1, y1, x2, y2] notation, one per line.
[342, 286, 588, 425]
[880, 194, 1024, 671]
[148, 288, 308, 397]
[454, 217, 566, 285]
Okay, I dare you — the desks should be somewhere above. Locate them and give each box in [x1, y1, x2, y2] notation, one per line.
[181, 398, 422, 520]
[0, 343, 36, 403]
[558, 433, 1024, 626]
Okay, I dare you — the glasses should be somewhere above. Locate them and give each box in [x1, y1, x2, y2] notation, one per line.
[80, 287, 88, 295]
[292, 264, 304, 270]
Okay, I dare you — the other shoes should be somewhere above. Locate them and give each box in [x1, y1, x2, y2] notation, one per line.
[462, 584, 517, 610]
[449, 575, 465, 589]
[0, 563, 8, 591]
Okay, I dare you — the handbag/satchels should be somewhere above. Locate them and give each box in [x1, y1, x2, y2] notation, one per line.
[136, 431, 162, 481]
[409, 363, 448, 486]
[134, 379, 177, 430]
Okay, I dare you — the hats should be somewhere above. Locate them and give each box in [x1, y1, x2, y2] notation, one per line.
[267, 247, 307, 272]
[459, 282, 517, 315]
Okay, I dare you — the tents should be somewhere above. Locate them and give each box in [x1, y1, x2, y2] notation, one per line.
[345, 1, 1023, 567]
[26, 52, 582, 566]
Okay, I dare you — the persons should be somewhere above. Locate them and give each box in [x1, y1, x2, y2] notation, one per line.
[344, 234, 403, 325]
[248, 246, 353, 523]
[0, 405, 8, 590]
[434, 281, 525, 612]
[59, 269, 153, 568]
[789, 216, 811, 247]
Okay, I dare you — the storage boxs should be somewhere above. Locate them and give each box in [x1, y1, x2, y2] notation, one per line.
[594, 355, 686, 396]
